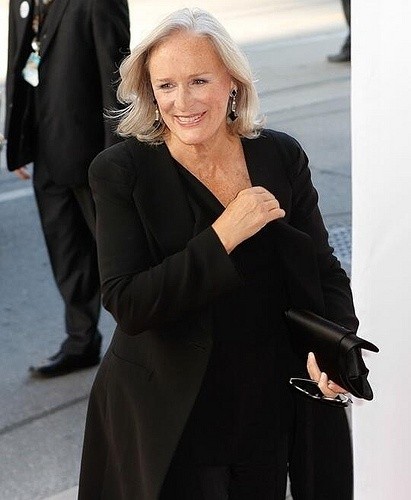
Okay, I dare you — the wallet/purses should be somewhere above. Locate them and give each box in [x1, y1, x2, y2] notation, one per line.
[285, 306, 380, 401]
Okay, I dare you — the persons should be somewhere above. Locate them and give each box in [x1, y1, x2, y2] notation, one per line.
[88, 9, 359, 500]
[5, 0, 132, 376]
[326, 0, 350, 62]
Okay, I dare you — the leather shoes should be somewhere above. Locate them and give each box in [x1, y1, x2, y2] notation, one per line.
[28, 351, 101, 377]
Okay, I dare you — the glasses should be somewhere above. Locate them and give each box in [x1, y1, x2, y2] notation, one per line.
[290, 378, 354, 407]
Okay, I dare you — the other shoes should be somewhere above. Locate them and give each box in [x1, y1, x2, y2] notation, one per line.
[328, 48, 352, 62]
